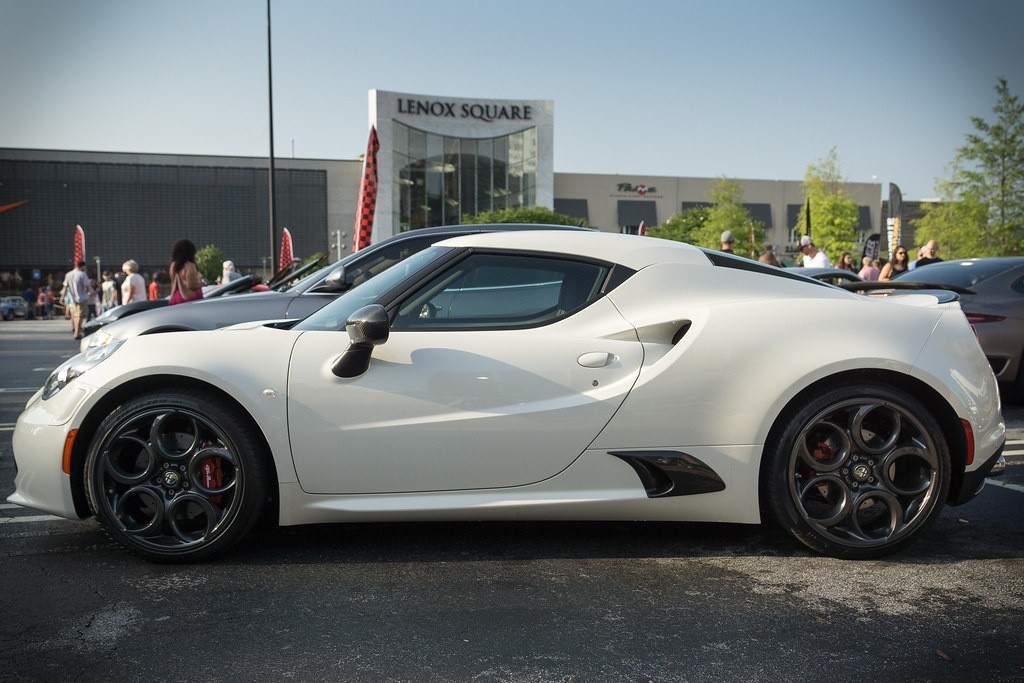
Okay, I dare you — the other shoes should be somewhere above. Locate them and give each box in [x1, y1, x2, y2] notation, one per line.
[75, 335, 83, 339]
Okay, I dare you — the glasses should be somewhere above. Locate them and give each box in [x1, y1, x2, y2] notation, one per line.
[898, 252, 906, 255]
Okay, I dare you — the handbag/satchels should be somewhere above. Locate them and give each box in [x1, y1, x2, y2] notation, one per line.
[168, 263, 203, 305]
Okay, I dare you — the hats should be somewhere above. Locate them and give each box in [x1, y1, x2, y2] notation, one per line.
[721, 231, 735, 242]
[801, 236, 813, 247]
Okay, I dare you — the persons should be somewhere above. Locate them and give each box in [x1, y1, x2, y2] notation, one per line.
[801, 235, 833, 268]
[720, 230, 735, 254]
[216, 275, 222, 284]
[759, 241, 781, 267]
[835, 252, 853, 272]
[121, 259, 147, 305]
[59, 260, 119, 339]
[149, 271, 163, 301]
[878, 245, 909, 281]
[23, 284, 56, 320]
[857, 256, 881, 282]
[908, 239, 945, 270]
[851, 259, 859, 275]
[169, 240, 207, 306]
[222, 260, 242, 285]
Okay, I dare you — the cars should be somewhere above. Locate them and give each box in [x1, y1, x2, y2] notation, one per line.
[0, 296, 29, 321]
[862, 255, 1024, 417]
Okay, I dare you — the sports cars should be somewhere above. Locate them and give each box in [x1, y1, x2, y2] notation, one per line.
[8, 229, 1008, 562]
[79, 224, 869, 354]
